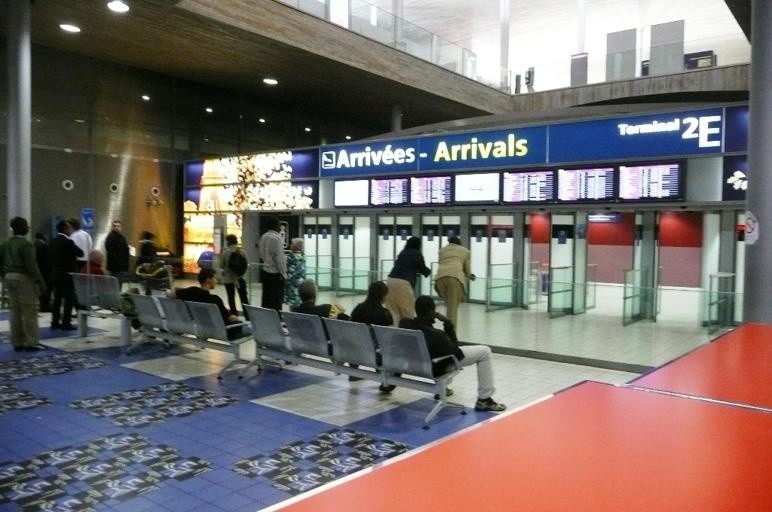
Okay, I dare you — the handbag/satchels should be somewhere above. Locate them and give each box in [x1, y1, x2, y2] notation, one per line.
[227, 250, 248, 277]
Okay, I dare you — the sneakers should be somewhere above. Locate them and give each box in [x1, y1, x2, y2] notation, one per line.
[51, 321, 77, 332]
[434, 387, 454, 400]
[14, 342, 49, 353]
[474, 397, 506, 412]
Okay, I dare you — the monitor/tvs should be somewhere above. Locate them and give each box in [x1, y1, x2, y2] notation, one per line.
[409, 173, 453, 205]
[453, 170, 501, 205]
[369, 175, 409, 206]
[333, 177, 369, 208]
[502, 166, 555, 204]
[615, 159, 686, 202]
[555, 162, 617, 203]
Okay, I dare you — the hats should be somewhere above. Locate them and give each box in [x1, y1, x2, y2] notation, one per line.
[291, 237, 305, 250]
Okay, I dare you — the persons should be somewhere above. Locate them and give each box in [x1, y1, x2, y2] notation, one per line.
[103, 219, 131, 274]
[163, 268, 254, 341]
[220, 233, 251, 322]
[257, 216, 287, 328]
[433, 236, 477, 331]
[80, 251, 143, 333]
[33, 232, 53, 314]
[0, 216, 49, 352]
[397, 295, 506, 412]
[288, 277, 364, 381]
[68, 216, 93, 318]
[140, 230, 157, 295]
[283, 235, 307, 312]
[47, 219, 85, 331]
[383, 235, 431, 324]
[349, 279, 401, 394]
